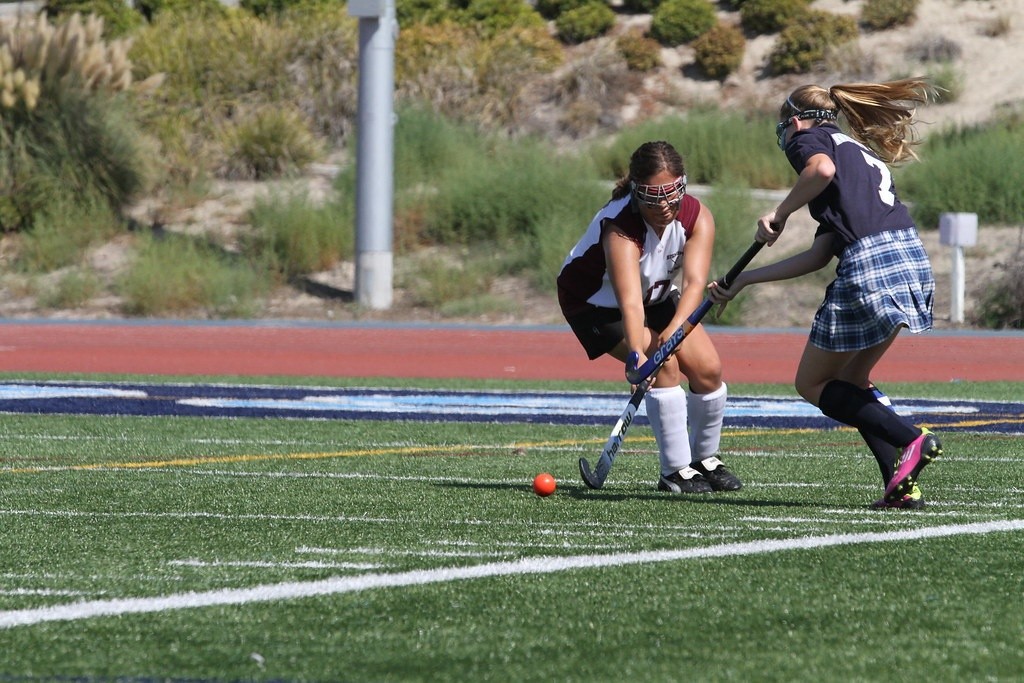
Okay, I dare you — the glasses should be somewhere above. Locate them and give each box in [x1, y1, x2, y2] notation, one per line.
[775, 123, 787, 151]
[630, 175, 687, 206]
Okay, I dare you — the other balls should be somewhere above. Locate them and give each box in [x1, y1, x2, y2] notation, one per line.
[532, 472, 557, 498]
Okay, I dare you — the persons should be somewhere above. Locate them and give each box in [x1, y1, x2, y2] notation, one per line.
[556, 142, 740, 495]
[709, 75, 942, 507]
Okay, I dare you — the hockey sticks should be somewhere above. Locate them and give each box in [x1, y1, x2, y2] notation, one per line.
[624, 222, 779, 386]
[578, 360, 666, 490]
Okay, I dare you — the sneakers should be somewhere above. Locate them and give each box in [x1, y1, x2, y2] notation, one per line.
[872, 484, 924, 509]
[657, 464, 712, 494]
[884, 426, 943, 504]
[690, 455, 742, 491]
